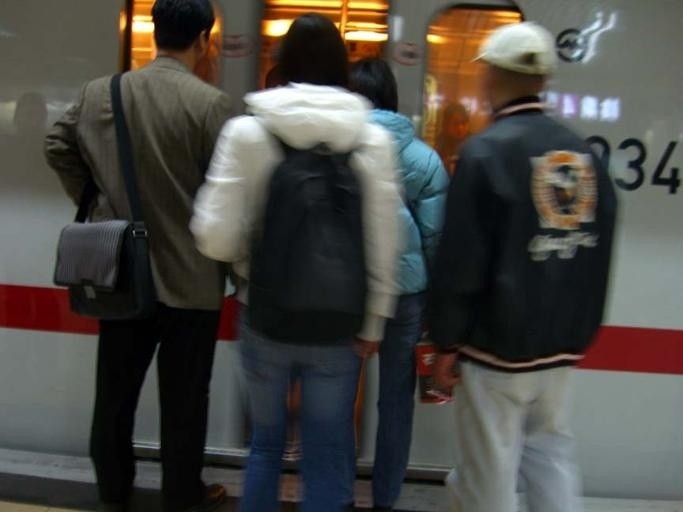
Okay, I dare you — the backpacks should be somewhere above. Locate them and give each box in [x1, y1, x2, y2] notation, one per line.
[249, 133, 368, 347]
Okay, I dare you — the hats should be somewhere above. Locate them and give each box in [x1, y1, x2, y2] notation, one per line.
[471, 23, 557, 78]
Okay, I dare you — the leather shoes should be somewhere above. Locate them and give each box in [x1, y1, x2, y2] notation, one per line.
[205, 483, 227, 505]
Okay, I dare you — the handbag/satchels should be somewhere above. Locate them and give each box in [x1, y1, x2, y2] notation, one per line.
[51, 220, 151, 320]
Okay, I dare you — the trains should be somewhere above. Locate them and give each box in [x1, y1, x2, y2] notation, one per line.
[0, 3, 681, 510]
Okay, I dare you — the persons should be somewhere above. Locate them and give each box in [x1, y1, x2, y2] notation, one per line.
[41, 0, 233, 512]
[429, 19, 618, 511]
[354, 58, 449, 512]
[189, 13, 402, 511]
[435, 101, 474, 176]
